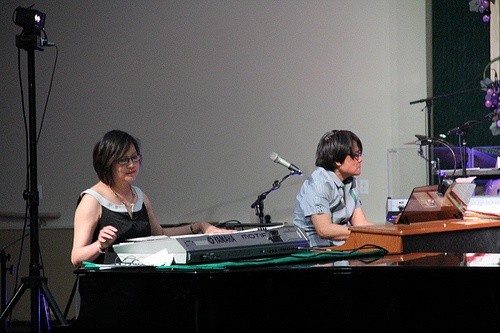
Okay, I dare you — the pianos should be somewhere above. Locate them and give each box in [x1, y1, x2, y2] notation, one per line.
[318, 209, 500, 254]
[433, 169, 500, 180]
[111, 222, 311, 267]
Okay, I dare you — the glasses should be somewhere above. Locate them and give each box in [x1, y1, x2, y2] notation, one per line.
[347, 148, 362, 159]
[112, 153, 142, 165]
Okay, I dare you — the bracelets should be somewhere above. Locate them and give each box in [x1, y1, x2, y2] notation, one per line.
[97, 241, 108, 253]
[189, 224, 199, 234]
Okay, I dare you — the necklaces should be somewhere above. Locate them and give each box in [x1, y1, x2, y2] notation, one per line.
[110, 183, 135, 207]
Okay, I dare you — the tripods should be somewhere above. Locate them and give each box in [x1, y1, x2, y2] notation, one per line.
[0, 36, 71, 333]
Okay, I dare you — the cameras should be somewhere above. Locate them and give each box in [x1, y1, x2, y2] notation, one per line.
[14, 8, 46, 29]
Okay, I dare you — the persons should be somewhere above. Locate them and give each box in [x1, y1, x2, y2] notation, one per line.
[295, 130, 375, 247]
[70, 130, 237, 268]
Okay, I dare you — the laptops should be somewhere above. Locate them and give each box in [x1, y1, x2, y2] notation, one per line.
[450, 184, 476, 205]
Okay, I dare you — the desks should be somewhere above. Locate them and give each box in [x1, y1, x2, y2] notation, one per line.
[72, 250, 500, 333]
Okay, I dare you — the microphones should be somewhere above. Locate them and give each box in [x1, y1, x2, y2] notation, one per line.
[270, 152, 302, 176]
[416, 138, 458, 194]
